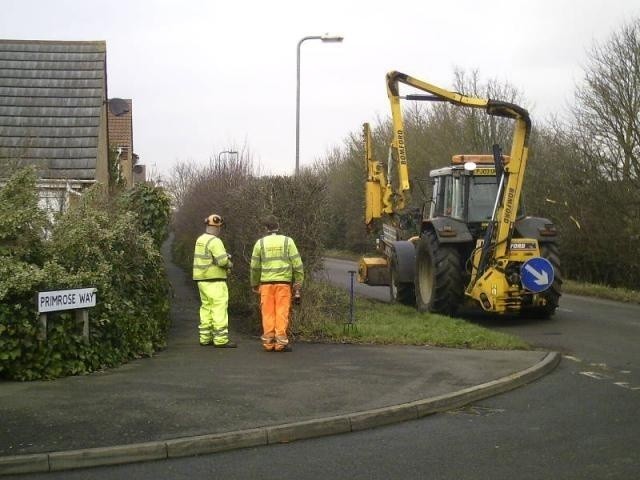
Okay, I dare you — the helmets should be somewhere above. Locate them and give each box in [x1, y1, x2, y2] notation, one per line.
[205, 214, 224, 226]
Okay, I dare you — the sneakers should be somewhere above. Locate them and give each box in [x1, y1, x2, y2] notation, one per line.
[275, 344, 292, 352]
[215, 340, 237, 348]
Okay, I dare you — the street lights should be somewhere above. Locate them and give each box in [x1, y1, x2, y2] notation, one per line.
[219, 152, 237, 176]
[296, 35, 344, 176]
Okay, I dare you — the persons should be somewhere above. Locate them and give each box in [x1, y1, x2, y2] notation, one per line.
[192, 214, 237, 349]
[250, 214, 304, 353]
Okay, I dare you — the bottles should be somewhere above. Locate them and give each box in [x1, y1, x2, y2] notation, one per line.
[294, 290, 301, 305]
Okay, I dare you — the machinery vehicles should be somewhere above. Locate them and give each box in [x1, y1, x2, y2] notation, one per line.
[358, 72, 562, 318]
